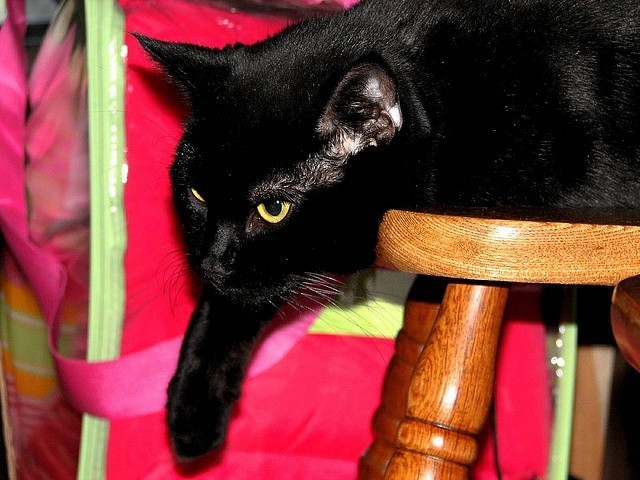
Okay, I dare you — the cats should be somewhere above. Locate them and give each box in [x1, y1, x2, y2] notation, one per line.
[122, 0, 640, 465]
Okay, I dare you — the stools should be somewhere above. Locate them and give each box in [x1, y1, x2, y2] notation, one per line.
[357, 209, 640, 480]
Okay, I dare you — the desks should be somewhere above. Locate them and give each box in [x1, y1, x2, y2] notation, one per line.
[611, 274, 640, 373]
